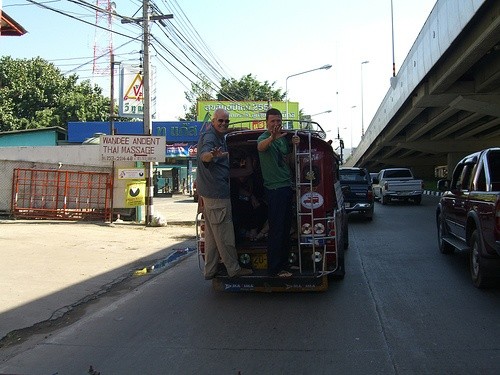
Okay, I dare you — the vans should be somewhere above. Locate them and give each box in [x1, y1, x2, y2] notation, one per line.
[194, 118, 352, 293]
[339, 166, 379, 222]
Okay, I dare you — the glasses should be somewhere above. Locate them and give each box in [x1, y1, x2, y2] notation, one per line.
[215, 118, 230, 123]
[278, 152, 283, 168]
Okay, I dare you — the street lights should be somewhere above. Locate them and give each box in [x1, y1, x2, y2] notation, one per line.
[286, 63, 332, 130]
[350, 105, 357, 155]
[360, 60, 370, 138]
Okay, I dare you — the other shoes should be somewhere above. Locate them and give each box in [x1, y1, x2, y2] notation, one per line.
[239, 268, 253, 275]
[205, 263, 223, 280]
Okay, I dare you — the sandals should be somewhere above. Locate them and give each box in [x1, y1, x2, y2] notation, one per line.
[254, 231, 270, 242]
[249, 230, 257, 243]
[277, 271, 293, 277]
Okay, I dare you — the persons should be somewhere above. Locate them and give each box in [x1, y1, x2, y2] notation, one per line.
[196, 109, 254, 281]
[257, 109, 301, 277]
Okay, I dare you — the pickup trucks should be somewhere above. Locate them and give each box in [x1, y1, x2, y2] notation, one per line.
[371, 167, 424, 207]
[435, 147, 500, 289]
